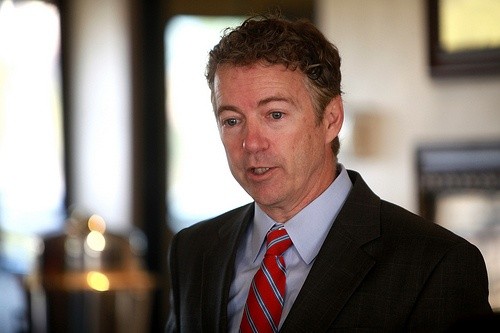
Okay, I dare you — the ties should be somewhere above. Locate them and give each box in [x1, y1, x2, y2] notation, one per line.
[238, 228, 294, 333]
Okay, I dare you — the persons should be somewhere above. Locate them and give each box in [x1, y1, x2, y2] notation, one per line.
[167, 10, 500, 333]
